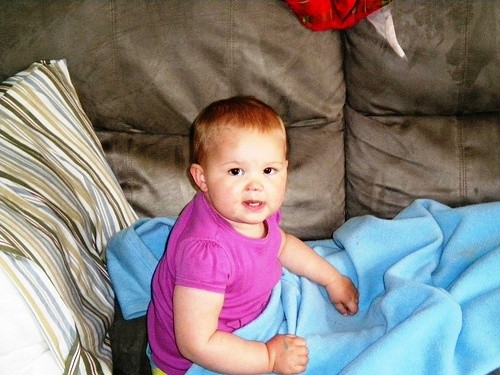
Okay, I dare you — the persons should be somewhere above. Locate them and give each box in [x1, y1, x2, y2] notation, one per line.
[147, 94, 360, 375]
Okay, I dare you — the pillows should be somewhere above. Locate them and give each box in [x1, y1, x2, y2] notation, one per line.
[0, 57, 139, 375]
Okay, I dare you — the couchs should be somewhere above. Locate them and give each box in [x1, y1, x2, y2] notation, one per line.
[0, 1, 499, 373]
